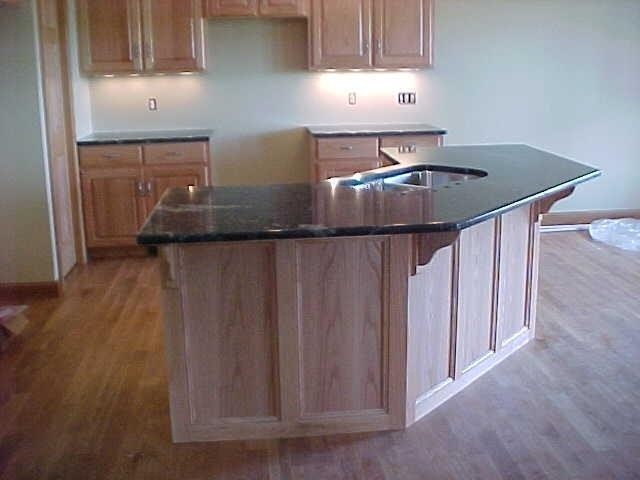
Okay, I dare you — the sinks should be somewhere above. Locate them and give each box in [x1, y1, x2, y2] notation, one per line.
[383, 163, 487, 187]
[337, 176, 425, 192]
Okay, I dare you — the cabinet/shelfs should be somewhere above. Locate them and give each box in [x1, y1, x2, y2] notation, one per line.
[200, 0, 310, 18]
[301, 122, 448, 185]
[78, 130, 214, 259]
[310, 185, 435, 231]
[74, 0, 206, 77]
[307, 1, 433, 71]
